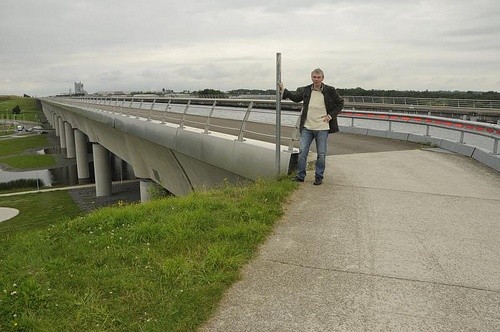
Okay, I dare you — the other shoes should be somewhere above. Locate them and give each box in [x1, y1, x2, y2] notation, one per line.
[314, 177, 323, 185]
[292, 176, 304, 182]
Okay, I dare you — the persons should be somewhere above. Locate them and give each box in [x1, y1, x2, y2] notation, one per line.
[277, 68, 344, 185]
